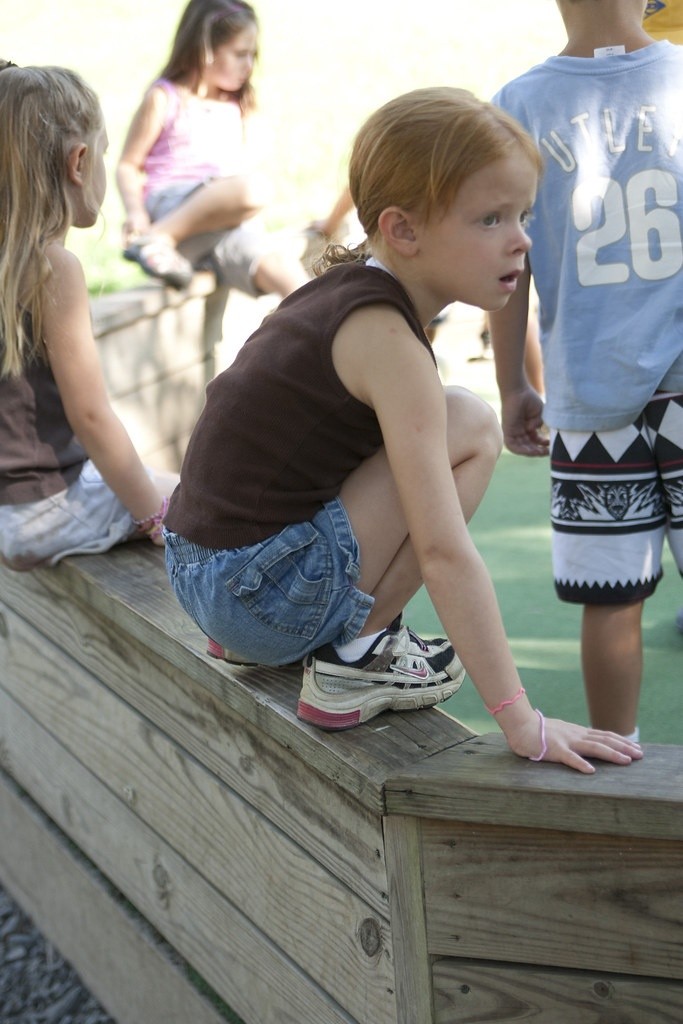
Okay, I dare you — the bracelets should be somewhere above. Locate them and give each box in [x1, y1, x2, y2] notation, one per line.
[529, 709, 548, 762]
[482, 688, 526, 714]
[128, 494, 170, 540]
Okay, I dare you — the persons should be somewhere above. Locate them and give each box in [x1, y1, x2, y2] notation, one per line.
[486, 0, 683, 747]
[303, 0, 683, 441]
[162, 86, 643, 774]
[113, 0, 309, 300]
[0, 58, 181, 568]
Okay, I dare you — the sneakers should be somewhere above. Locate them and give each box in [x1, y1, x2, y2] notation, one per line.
[206, 635, 305, 672]
[296, 610, 468, 730]
[122, 231, 192, 289]
